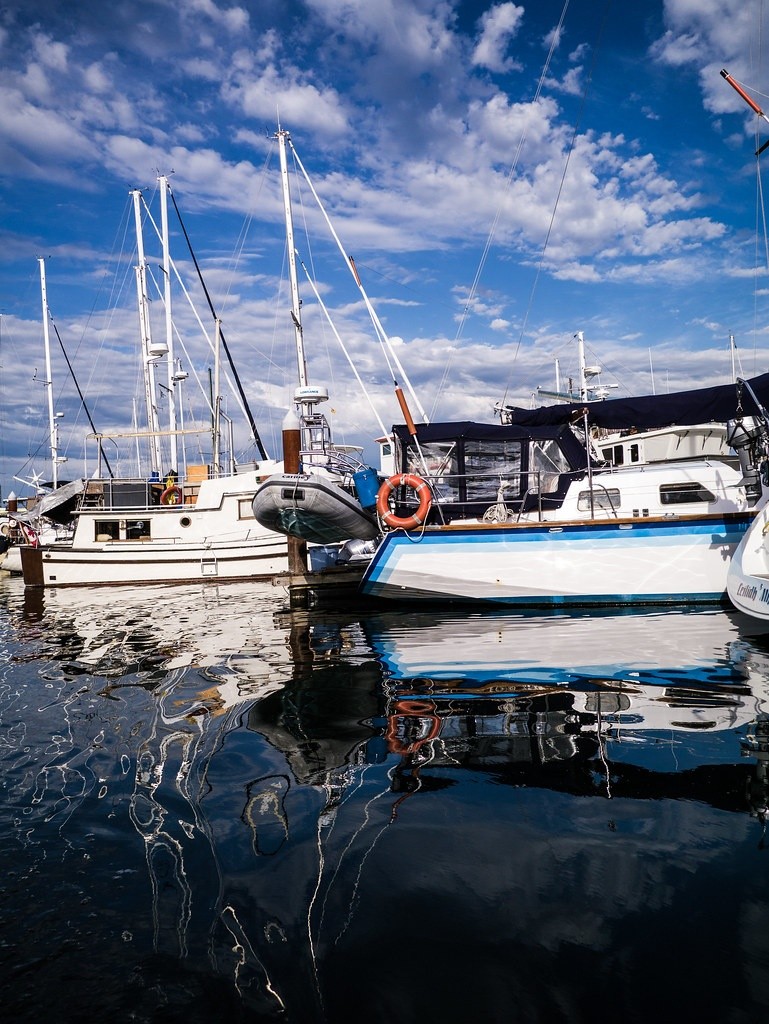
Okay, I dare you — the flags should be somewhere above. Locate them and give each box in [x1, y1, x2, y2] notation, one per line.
[21, 523, 37, 546]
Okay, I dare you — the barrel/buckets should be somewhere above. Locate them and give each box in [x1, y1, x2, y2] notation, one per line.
[353, 469, 379, 508]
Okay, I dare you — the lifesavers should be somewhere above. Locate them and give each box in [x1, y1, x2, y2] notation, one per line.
[159, 486, 185, 508]
[376, 473, 430, 531]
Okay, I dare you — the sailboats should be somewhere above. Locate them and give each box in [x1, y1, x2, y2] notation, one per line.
[0, 105, 769, 619]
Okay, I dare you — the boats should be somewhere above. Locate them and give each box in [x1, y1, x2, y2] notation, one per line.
[252, 472, 380, 547]
[354, 600, 768, 692]
